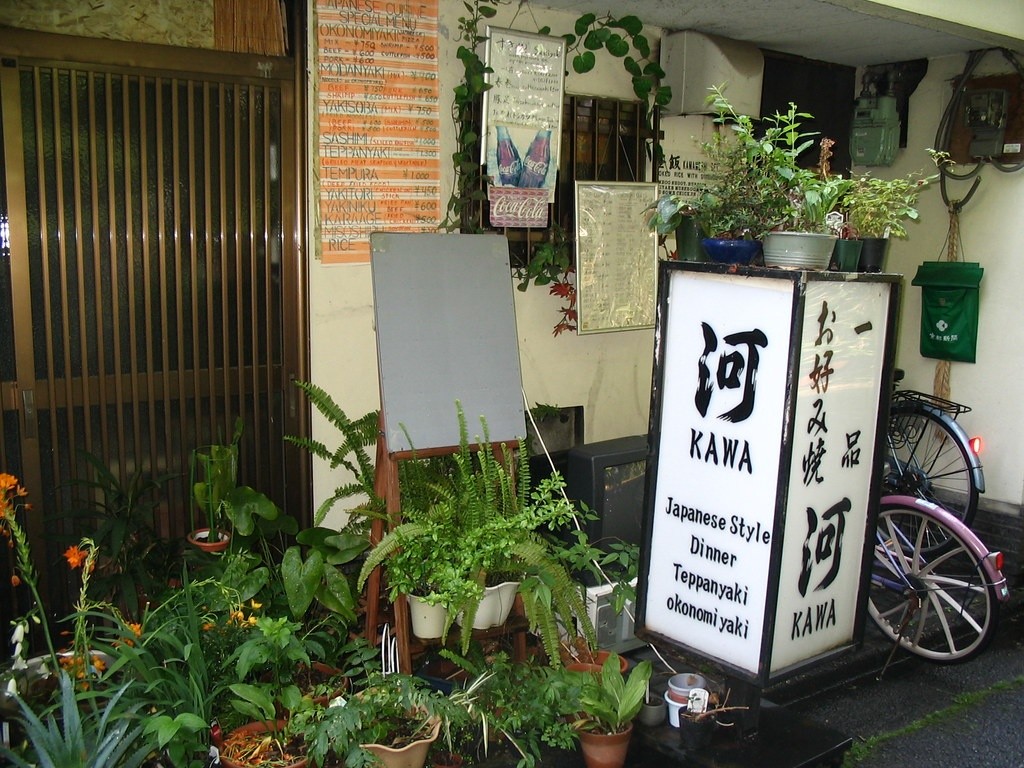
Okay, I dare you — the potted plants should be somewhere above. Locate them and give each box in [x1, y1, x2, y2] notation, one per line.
[0, 380, 652, 768]
[643, 80, 957, 272]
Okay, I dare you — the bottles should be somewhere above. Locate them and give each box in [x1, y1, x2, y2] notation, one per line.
[495, 126, 523, 188]
[517, 129, 552, 189]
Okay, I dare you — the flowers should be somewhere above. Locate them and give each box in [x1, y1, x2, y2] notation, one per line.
[0, 474, 142, 692]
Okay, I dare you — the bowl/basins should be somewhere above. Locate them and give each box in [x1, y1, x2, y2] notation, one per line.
[664, 672, 708, 727]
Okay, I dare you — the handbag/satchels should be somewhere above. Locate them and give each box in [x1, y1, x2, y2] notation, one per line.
[910, 199, 985, 364]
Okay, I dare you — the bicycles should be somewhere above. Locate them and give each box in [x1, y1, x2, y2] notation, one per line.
[873, 368, 988, 559]
[867, 492, 1011, 684]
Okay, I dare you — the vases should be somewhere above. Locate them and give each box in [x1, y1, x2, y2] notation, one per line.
[0, 651, 124, 720]
[678, 706, 719, 750]
